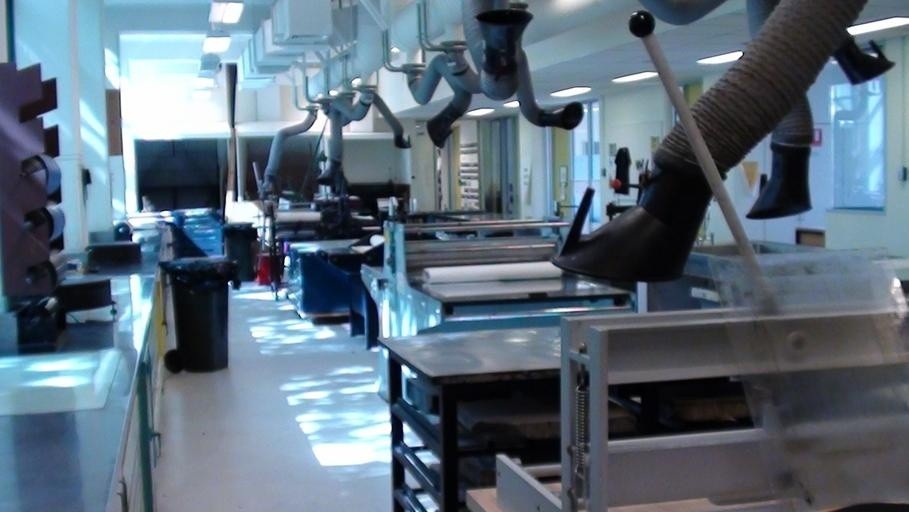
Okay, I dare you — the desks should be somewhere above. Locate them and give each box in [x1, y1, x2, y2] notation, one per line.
[378, 325, 561, 512]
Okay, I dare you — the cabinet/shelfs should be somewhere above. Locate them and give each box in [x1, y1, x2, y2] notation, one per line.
[0, 215, 174, 512]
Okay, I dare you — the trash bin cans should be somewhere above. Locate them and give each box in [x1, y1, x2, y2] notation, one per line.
[221, 221, 260, 281]
[159, 258, 233, 374]
